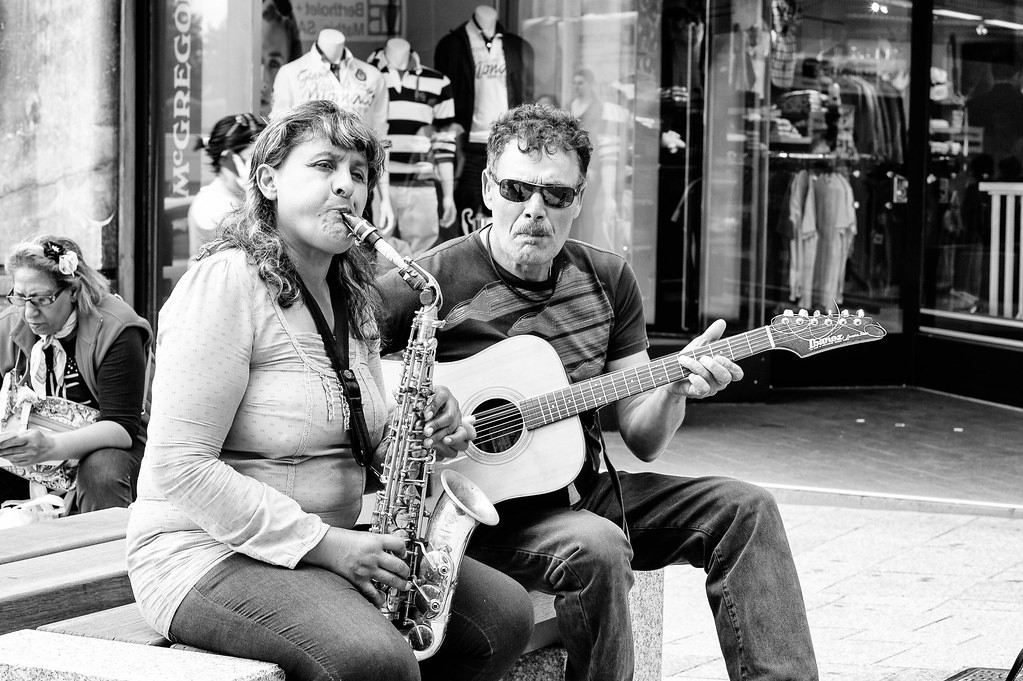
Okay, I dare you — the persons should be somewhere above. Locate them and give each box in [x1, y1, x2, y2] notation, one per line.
[270, 28, 396, 238]
[434, 8, 534, 241]
[365, 38, 457, 257]
[0, 236, 153, 515]
[973, 63, 1023, 317]
[370, 103, 818, 681]
[188, 114, 268, 269]
[127, 101, 533, 681]
[260, 0, 302, 118]
[536, 70, 602, 243]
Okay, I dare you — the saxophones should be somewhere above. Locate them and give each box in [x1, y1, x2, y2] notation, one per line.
[341, 210, 501, 663]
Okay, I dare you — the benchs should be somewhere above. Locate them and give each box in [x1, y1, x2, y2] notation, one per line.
[0, 503, 665, 681]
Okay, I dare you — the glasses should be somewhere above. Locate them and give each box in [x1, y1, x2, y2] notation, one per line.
[486, 171, 588, 208]
[6, 284, 70, 306]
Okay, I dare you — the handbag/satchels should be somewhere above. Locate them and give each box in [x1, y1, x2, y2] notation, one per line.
[0, 369, 99, 492]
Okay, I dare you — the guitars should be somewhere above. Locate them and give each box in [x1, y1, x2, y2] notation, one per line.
[354, 308, 888, 527]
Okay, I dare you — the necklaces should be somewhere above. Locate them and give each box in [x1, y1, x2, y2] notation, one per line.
[486, 225, 559, 302]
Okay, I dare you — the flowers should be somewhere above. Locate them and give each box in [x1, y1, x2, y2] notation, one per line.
[41, 240, 79, 278]
